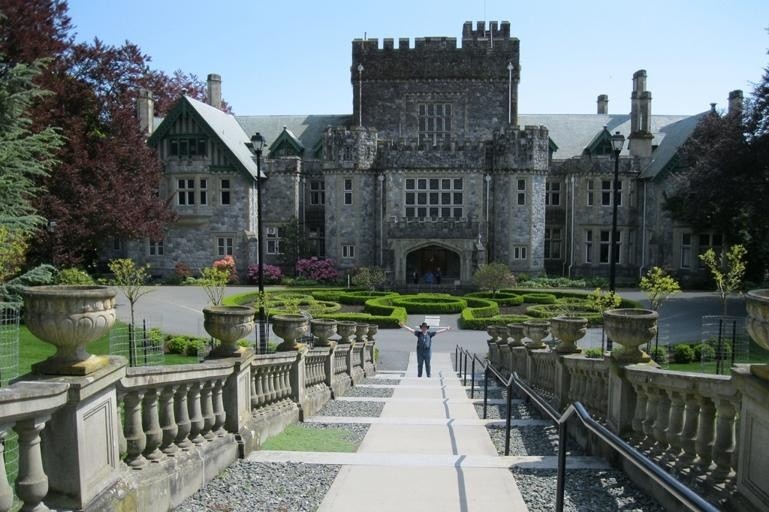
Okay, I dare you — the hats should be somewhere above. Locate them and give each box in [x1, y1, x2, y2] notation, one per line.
[419, 322, 429, 329]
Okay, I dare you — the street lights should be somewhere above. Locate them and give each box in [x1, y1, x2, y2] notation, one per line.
[250, 132, 265, 323]
[610, 130, 625, 291]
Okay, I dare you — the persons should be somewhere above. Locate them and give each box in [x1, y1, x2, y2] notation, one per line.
[397, 321, 453, 378]
[423, 270, 436, 284]
[411, 268, 419, 285]
[434, 266, 442, 284]
